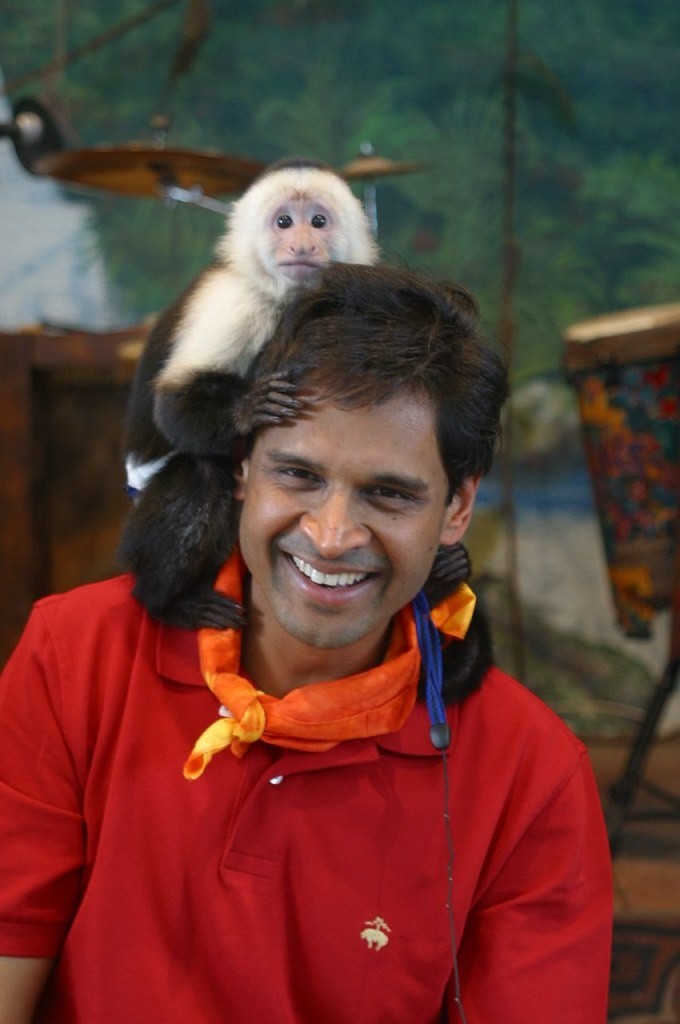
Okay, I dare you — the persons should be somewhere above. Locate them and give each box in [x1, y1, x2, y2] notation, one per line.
[1, 261, 614, 1024]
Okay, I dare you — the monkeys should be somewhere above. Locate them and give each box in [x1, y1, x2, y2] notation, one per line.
[109, 153, 494, 707]
